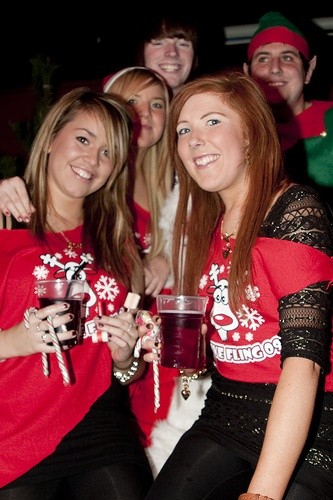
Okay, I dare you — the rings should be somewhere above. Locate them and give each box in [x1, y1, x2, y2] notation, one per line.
[36, 321, 42, 331]
[32, 309, 39, 320]
[40, 334, 48, 344]
[128, 322, 133, 331]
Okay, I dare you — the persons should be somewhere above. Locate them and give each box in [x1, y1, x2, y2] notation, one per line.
[236, 11, 332, 188]
[0, 87, 156, 500]
[133, 9, 196, 93]
[139, 72, 333, 500]
[0, 65, 214, 479]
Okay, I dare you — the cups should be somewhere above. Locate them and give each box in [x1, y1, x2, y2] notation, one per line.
[154, 295, 208, 369]
[36, 280, 85, 346]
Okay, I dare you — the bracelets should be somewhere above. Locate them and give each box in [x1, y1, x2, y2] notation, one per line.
[112, 361, 138, 383]
[237, 493, 275, 500]
[114, 359, 136, 371]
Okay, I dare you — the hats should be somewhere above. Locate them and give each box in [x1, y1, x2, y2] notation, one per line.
[247, 11, 308, 63]
[101, 66, 174, 110]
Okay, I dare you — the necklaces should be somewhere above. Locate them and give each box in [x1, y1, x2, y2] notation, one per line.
[218, 212, 243, 258]
[40, 209, 85, 253]
